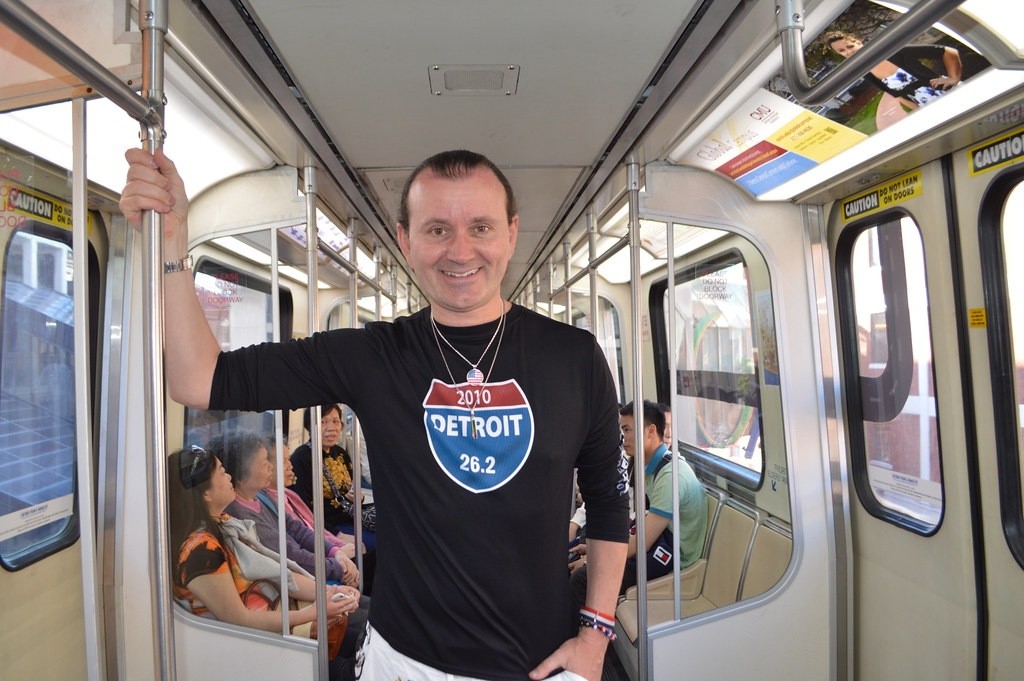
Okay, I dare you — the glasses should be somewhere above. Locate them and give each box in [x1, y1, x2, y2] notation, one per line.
[189, 444, 206, 477]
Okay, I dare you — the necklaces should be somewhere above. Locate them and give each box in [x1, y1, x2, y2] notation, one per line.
[431, 295, 503, 386]
[430, 300, 506, 438]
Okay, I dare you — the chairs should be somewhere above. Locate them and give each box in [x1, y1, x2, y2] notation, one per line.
[613, 481, 793, 647]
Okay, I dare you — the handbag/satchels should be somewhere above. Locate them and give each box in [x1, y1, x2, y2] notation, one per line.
[336, 495, 376, 533]
[630, 511, 675, 581]
[239, 579, 348, 660]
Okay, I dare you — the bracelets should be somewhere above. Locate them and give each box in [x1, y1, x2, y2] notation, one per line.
[164, 255, 193, 273]
[579, 605, 619, 629]
[580, 618, 618, 643]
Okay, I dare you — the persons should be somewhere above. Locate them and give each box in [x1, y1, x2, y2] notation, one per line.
[825, 31, 963, 110]
[166, 401, 377, 681]
[119, 146, 631, 681]
[566, 400, 707, 609]
